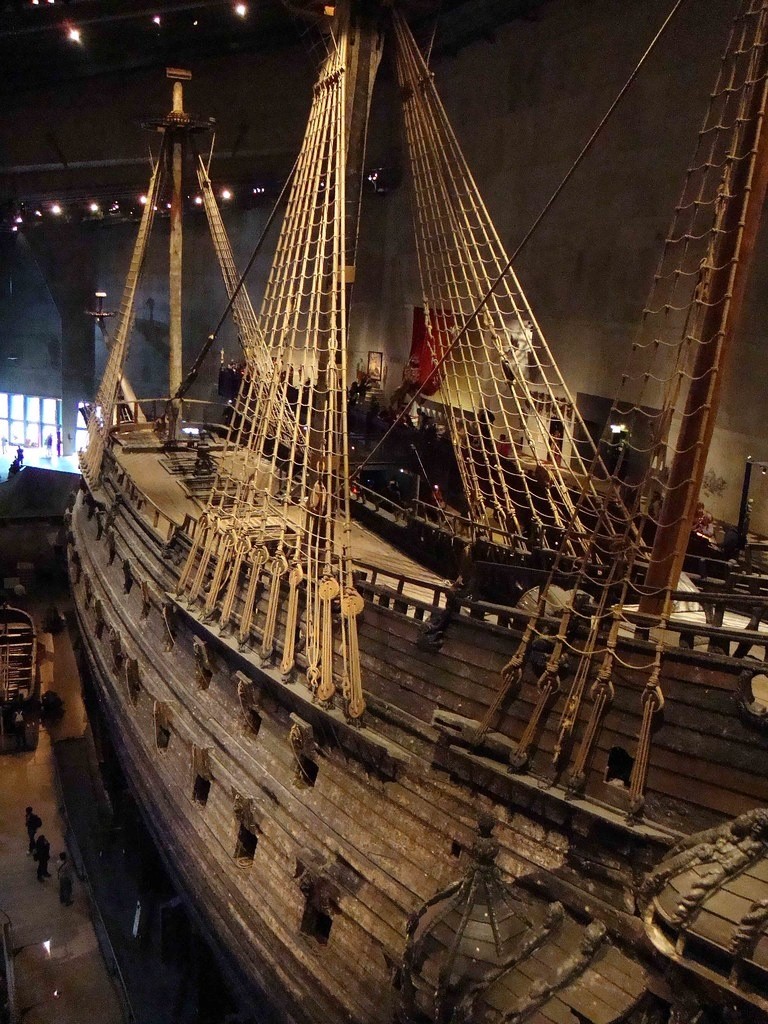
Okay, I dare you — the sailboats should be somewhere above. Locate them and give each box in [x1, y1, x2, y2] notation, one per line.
[63, 1, 768, 1024]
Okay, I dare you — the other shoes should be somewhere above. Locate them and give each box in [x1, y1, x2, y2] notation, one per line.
[61, 899, 74, 906]
[26, 842, 35, 856]
[37, 871, 51, 882]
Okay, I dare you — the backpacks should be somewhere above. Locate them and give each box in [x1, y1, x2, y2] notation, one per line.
[32, 813, 42, 828]
[14, 710, 24, 723]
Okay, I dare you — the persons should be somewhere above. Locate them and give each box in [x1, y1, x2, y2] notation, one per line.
[45, 433, 54, 458]
[624, 479, 663, 517]
[351, 379, 513, 519]
[25, 806, 41, 854]
[1, 435, 7, 455]
[34, 834, 51, 882]
[16, 446, 25, 464]
[6, 455, 18, 479]
[56, 852, 76, 907]
[693, 511, 719, 538]
